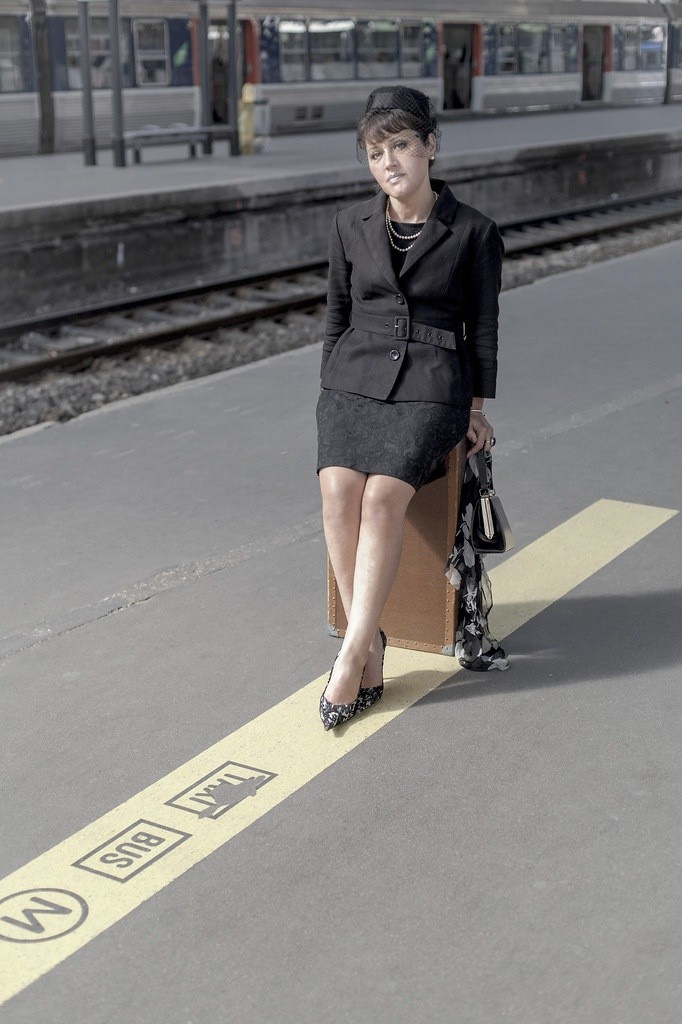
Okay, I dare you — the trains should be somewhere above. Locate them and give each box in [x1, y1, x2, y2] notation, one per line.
[0, 1, 680, 163]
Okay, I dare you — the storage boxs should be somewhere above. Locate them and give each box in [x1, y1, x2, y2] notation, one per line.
[327, 436, 470, 656]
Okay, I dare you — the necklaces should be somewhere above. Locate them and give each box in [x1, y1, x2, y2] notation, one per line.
[383, 196, 417, 252]
[385, 191, 441, 239]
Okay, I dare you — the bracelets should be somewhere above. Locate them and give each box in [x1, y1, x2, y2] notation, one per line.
[471, 410, 486, 416]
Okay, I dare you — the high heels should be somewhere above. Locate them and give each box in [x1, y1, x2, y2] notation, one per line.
[319, 649, 361, 731]
[353, 625, 388, 713]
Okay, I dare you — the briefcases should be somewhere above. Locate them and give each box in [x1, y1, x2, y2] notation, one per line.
[326, 433, 480, 656]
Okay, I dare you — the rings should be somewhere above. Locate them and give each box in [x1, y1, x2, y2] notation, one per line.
[485, 438, 496, 447]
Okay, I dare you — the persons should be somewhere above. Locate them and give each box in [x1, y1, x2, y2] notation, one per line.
[313, 88, 503, 732]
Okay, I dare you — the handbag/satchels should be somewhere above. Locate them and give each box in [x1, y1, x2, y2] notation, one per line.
[471, 490, 515, 554]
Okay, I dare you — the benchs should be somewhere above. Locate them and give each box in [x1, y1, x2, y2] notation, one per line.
[123, 125, 231, 163]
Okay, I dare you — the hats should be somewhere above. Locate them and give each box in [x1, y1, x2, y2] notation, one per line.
[355, 85, 441, 164]
